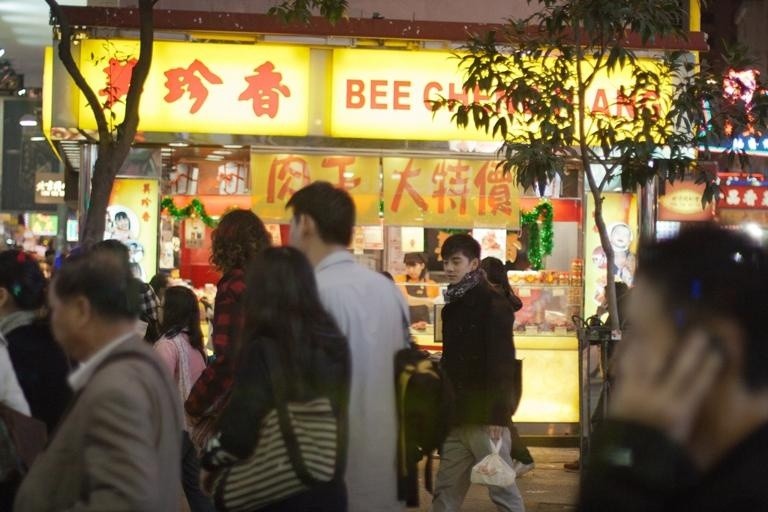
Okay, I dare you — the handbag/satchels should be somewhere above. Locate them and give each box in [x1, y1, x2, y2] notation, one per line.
[390, 343, 445, 507]
[210, 393, 349, 511]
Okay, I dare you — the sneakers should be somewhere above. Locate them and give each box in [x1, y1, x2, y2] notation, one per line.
[563, 461, 579, 473]
[513, 460, 535, 479]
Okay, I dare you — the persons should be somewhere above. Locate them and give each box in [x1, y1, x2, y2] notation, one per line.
[396, 253, 439, 324]
[430, 233, 534, 512]
[2, 239, 210, 512]
[184, 181, 415, 511]
[573, 217, 767, 511]
[563, 280, 629, 471]
